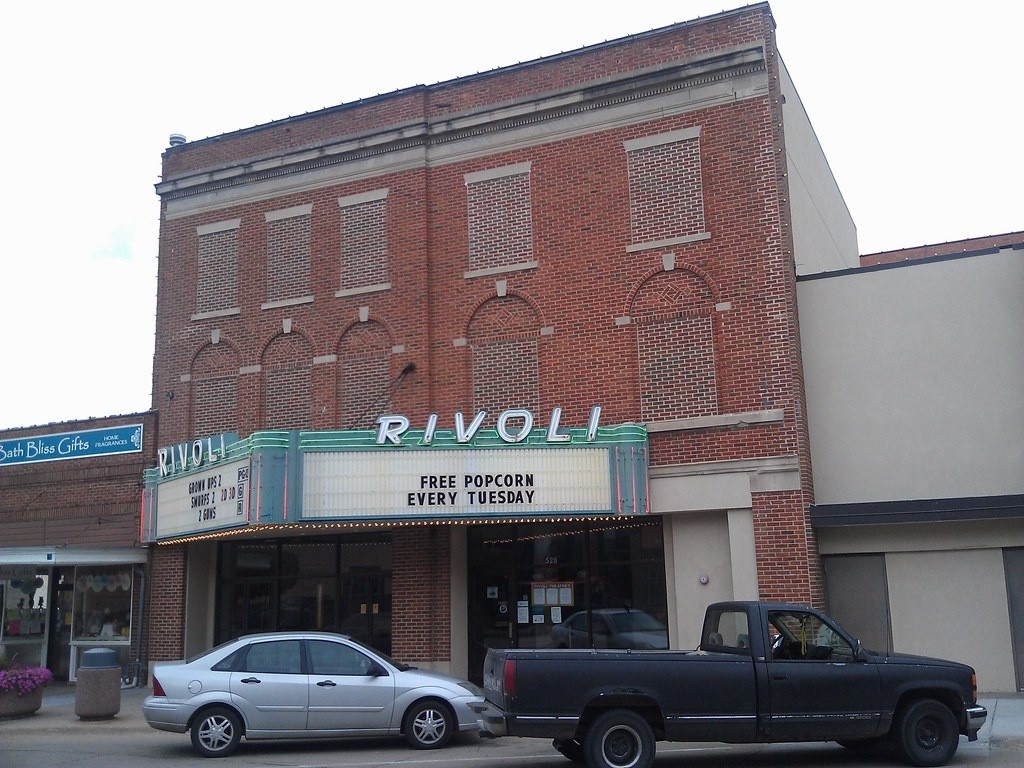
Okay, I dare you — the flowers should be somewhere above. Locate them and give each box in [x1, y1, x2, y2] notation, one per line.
[0, 653, 53, 695]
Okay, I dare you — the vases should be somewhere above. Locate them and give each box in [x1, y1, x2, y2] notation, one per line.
[0, 688, 42, 720]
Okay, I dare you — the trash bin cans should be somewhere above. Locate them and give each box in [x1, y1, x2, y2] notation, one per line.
[74, 648, 122, 721]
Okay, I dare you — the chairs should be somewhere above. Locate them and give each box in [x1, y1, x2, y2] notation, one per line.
[708, 632, 723, 647]
[735, 634, 750, 649]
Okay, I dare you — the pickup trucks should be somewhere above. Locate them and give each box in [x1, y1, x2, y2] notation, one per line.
[465, 600, 989, 768]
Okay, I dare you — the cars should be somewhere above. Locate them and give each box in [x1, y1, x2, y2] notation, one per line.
[141, 630, 486, 758]
[549, 606, 669, 651]
